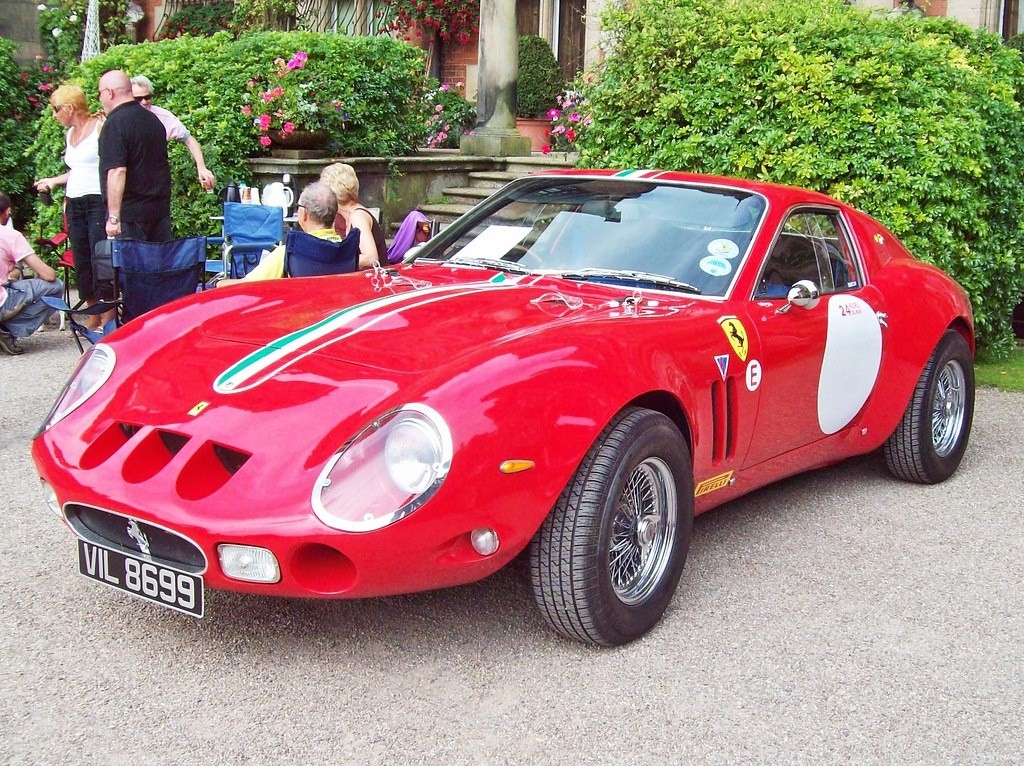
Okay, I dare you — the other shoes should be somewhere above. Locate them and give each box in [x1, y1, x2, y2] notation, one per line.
[0, 328, 23, 355]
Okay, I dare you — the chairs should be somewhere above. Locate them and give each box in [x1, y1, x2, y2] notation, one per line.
[34, 200, 441, 355]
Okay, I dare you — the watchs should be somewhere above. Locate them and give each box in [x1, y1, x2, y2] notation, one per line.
[108, 216, 121, 224]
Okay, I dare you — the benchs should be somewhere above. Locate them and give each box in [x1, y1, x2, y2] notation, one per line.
[561, 221, 847, 300]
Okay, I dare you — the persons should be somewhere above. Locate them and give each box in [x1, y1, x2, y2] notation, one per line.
[0, 192, 65, 356]
[131, 75, 214, 188]
[34, 86, 119, 337]
[98, 69, 172, 244]
[319, 162, 388, 270]
[215, 182, 343, 290]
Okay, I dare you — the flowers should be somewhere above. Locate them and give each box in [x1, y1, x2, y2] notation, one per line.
[419, 81, 477, 149]
[239, 50, 349, 152]
[541, 91, 593, 155]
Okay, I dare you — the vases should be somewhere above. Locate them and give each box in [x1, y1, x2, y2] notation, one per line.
[440, 140, 457, 149]
[266, 128, 330, 150]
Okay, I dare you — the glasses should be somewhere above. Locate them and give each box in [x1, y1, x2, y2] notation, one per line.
[134, 94, 151, 102]
[53, 105, 61, 112]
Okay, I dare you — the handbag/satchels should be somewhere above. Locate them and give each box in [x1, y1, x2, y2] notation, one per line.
[95, 238, 132, 280]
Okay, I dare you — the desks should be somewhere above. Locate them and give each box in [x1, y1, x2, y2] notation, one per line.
[209, 216, 299, 260]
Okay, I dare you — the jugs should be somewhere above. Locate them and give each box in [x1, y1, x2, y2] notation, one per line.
[217, 173, 299, 218]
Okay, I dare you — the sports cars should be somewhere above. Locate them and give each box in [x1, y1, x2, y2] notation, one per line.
[30, 168, 976, 648]
